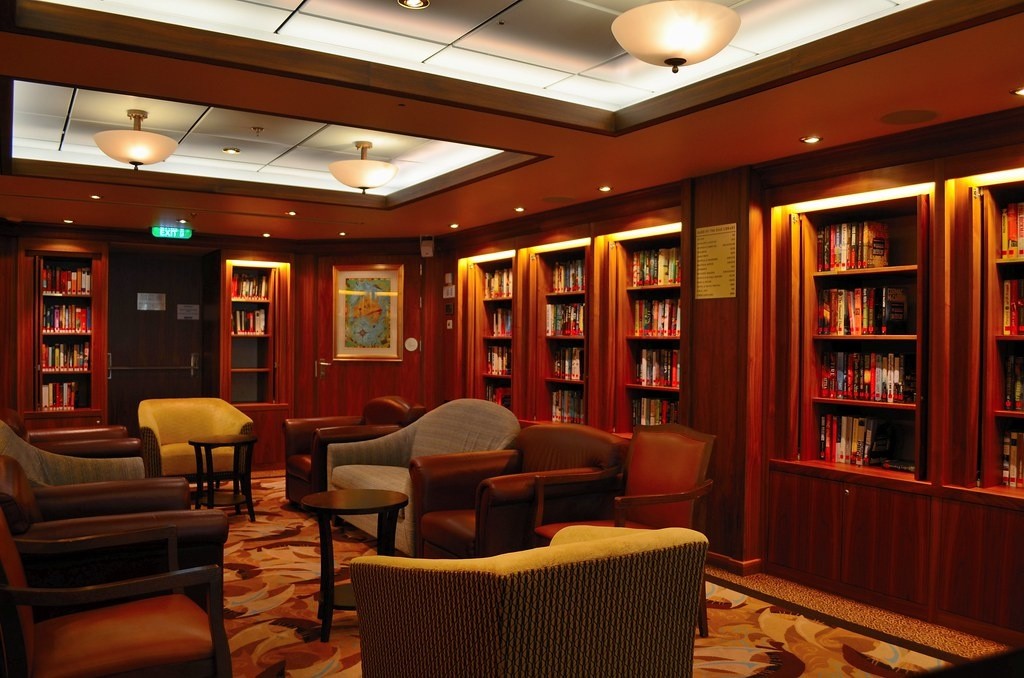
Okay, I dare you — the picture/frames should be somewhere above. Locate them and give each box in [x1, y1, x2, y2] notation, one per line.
[332, 265, 404, 362]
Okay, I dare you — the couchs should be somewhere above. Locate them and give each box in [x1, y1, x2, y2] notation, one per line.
[138, 398, 253, 483]
[350, 525, 709, 678]
[328, 398, 520, 557]
[0, 455, 229, 615]
[283, 396, 427, 507]
[409, 424, 630, 559]
[0, 420, 145, 487]
[0, 408, 142, 458]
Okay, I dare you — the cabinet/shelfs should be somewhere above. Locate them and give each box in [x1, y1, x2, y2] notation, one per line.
[0, 132, 1024, 648]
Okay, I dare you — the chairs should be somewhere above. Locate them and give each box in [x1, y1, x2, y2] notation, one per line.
[0, 512, 231, 678]
[532, 423, 718, 549]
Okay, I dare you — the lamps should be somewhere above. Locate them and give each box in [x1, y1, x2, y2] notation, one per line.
[611, 0, 741, 73]
[327, 141, 396, 194]
[92, 109, 178, 170]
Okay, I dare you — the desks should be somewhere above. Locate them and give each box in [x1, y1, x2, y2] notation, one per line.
[188, 434, 257, 522]
[300, 489, 409, 642]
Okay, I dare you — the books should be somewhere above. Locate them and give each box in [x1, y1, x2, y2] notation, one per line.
[42, 265, 91, 412]
[483, 266, 513, 412]
[632, 248, 681, 431]
[231, 272, 268, 336]
[818, 222, 915, 474]
[547, 260, 587, 429]
[998, 200, 1024, 491]
